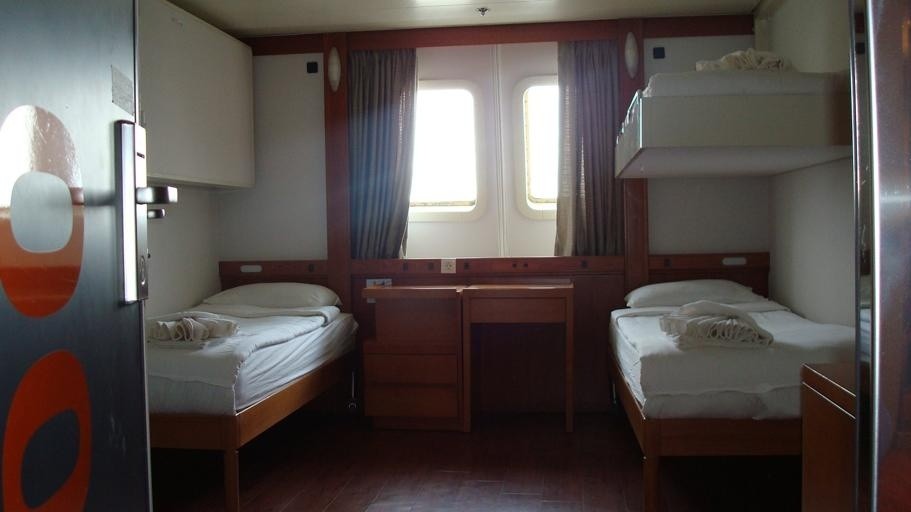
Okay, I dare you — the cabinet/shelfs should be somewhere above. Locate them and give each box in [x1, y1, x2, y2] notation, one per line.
[361, 284, 466, 434]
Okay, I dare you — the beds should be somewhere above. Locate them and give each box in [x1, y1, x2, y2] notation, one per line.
[614, 71, 853, 181]
[144, 279, 360, 512]
[607, 283, 857, 511]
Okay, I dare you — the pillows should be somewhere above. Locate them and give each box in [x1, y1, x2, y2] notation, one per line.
[203, 281, 342, 309]
[625, 278, 748, 307]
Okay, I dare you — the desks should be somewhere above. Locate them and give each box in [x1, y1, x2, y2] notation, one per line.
[462, 283, 576, 435]
[801, 364, 857, 512]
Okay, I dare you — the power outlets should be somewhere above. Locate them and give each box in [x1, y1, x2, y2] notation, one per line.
[440, 259, 456, 274]
